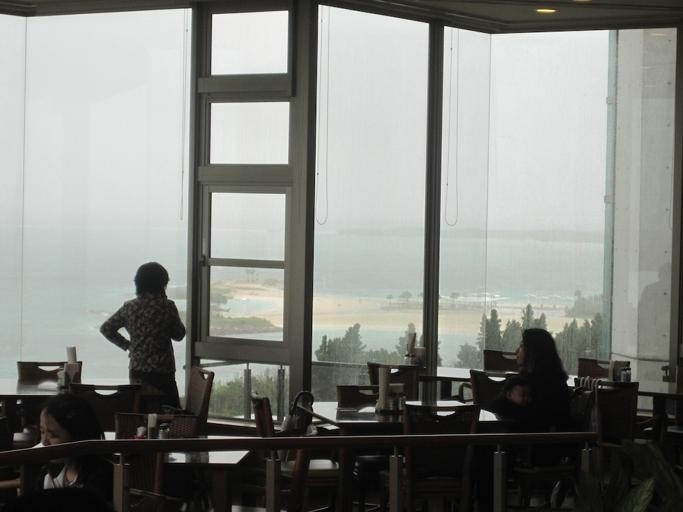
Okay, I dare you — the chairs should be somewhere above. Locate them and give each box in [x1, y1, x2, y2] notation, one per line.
[0, 349, 683, 512]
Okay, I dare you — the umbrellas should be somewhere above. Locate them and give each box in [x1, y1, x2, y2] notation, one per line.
[276, 391, 315, 467]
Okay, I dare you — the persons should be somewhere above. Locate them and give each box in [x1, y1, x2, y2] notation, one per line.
[483, 375, 533, 416]
[98, 261, 186, 415]
[470, 327, 570, 512]
[0, 393, 108, 500]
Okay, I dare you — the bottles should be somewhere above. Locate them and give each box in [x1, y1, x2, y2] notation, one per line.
[385, 383, 407, 415]
